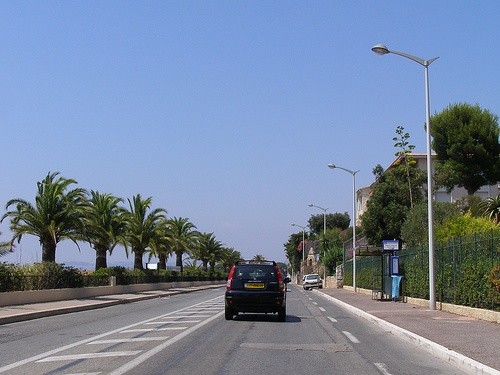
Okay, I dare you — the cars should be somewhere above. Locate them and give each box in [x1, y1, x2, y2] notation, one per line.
[301, 274, 323, 291]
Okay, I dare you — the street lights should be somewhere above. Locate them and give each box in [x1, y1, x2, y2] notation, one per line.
[291, 223, 307, 276]
[371, 43, 441, 311]
[328, 162, 360, 294]
[308, 204, 329, 288]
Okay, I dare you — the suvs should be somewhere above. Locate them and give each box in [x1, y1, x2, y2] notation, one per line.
[224, 259, 292, 322]
